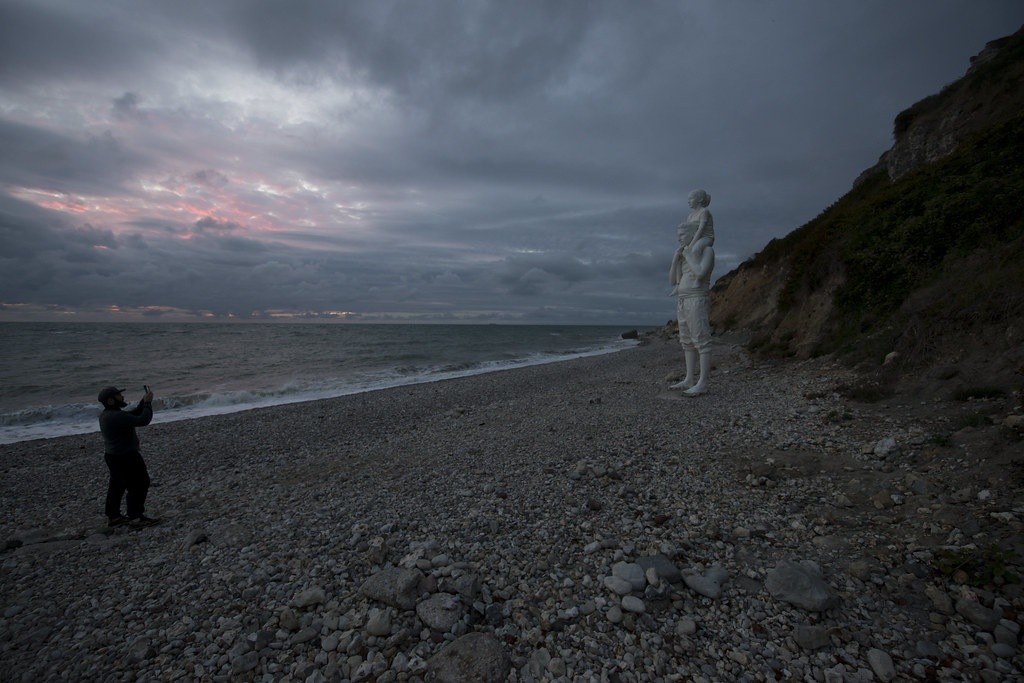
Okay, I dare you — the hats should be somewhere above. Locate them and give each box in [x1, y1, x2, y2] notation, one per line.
[98, 386, 126, 402]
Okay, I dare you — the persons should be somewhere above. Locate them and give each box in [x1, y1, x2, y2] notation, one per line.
[98, 386, 160, 528]
[668, 189, 715, 395]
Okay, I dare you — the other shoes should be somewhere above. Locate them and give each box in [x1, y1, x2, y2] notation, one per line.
[129, 515, 162, 530]
[108, 513, 129, 529]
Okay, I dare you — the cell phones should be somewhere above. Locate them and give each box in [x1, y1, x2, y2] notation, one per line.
[143, 385, 150, 393]
